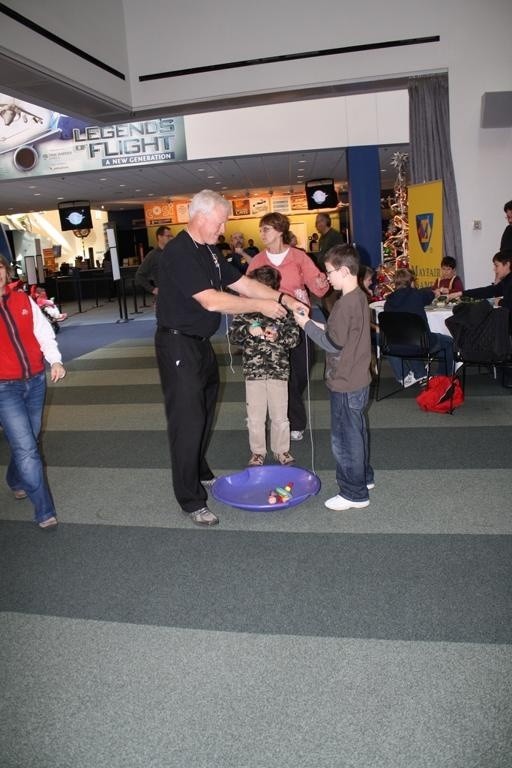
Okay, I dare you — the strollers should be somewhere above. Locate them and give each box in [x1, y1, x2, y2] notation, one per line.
[21, 282, 68, 334]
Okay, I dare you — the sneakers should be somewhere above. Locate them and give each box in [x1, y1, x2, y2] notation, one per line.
[290, 430, 304, 441]
[402, 371, 427, 388]
[183, 509, 219, 527]
[201, 476, 217, 488]
[14, 489, 27, 499]
[248, 453, 264, 466]
[60, 313, 68, 319]
[39, 517, 58, 530]
[275, 453, 296, 467]
[324, 495, 370, 511]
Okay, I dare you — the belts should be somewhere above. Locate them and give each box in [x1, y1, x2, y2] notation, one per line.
[159, 328, 203, 340]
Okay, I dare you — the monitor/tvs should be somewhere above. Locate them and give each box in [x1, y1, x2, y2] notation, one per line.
[305, 184, 337, 209]
[58, 206, 93, 231]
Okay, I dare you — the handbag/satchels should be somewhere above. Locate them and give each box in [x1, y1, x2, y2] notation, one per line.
[418, 376, 464, 411]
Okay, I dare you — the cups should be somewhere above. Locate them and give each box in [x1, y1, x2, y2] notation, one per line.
[13, 146, 38, 171]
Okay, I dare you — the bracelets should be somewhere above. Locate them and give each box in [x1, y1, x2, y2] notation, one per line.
[278, 292, 284, 303]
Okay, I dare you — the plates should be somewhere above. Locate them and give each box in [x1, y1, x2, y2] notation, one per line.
[212, 464, 322, 513]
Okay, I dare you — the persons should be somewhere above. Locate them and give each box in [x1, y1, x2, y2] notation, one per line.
[309, 233, 318, 251]
[292, 242, 377, 512]
[103, 250, 112, 273]
[495, 199, 512, 285]
[357, 252, 512, 388]
[134, 226, 173, 296]
[237, 212, 331, 443]
[150, 189, 311, 527]
[30, 284, 67, 322]
[225, 263, 300, 468]
[315, 212, 344, 316]
[216, 232, 259, 274]
[0, 253, 67, 533]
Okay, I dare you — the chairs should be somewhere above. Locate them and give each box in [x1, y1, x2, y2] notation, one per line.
[377, 302, 512, 415]
